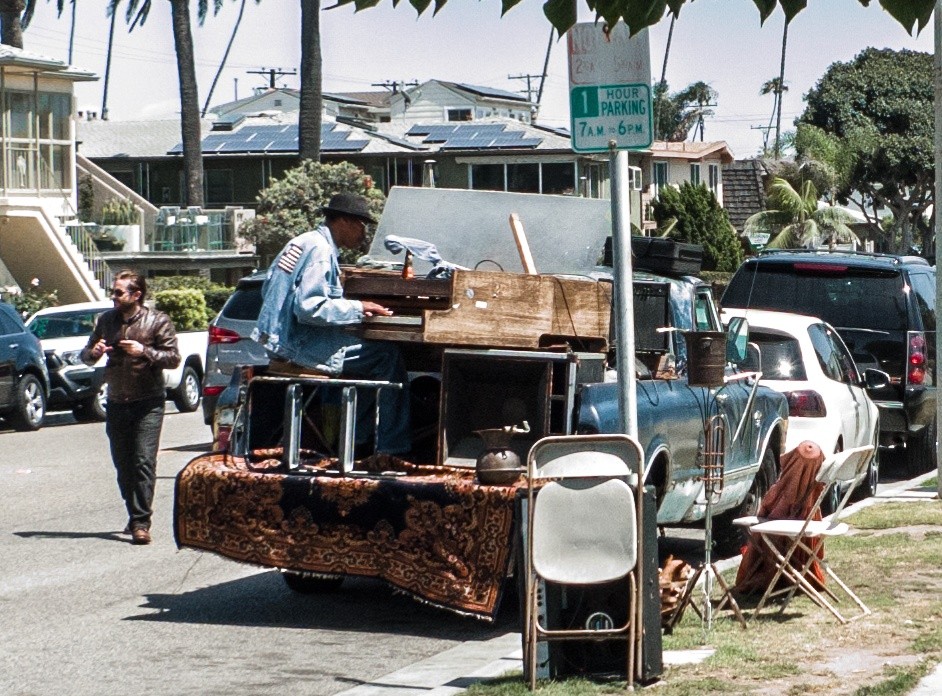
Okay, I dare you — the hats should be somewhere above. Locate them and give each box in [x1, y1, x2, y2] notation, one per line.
[320, 194, 379, 225]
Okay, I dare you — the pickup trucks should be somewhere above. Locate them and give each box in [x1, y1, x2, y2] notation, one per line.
[211, 235, 790, 593]
[24, 301, 209, 422]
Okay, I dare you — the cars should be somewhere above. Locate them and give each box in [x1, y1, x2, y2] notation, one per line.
[697, 307, 892, 514]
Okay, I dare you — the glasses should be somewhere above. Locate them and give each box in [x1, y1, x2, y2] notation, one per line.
[110, 288, 128, 297]
[360, 220, 370, 230]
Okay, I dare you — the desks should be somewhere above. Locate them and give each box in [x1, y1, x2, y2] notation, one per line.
[154, 214, 223, 249]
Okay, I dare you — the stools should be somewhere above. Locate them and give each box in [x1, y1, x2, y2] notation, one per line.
[268, 369, 332, 459]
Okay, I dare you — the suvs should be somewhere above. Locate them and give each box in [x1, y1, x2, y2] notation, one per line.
[201, 270, 272, 437]
[721, 249, 936, 480]
[0, 301, 50, 431]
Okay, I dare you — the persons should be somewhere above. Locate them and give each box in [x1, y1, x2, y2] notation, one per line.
[251, 192, 427, 464]
[79, 272, 182, 543]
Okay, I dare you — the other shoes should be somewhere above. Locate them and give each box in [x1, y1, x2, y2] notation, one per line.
[132, 528, 150, 542]
[124, 519, 133, 535]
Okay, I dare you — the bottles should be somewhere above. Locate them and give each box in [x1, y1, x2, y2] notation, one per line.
[474, 427, 520, 484]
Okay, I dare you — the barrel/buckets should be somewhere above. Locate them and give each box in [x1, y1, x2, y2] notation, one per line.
[680, 330, 727, 387]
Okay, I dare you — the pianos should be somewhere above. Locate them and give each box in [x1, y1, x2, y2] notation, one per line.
[338, 249, 612, 353]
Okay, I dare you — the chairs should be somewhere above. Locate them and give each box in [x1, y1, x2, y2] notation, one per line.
[152, 205, 244, 253]
[523, 432, 646, 696]
[732, 443, 876, 625]
[242, 371, 407, 480]
